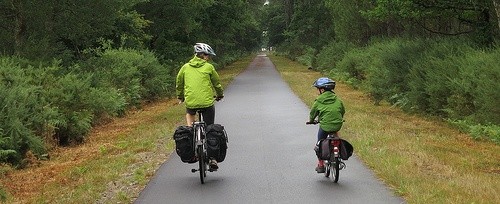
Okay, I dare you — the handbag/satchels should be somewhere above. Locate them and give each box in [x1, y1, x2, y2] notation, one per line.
[174, 126, 197, 163]
[341, 140, 353, 160]
[314, 140, 323, 160]
[206, 124, 228, 161]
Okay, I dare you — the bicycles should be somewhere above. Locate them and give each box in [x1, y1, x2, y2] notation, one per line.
[306, 120, 346, 183]
[177, 96, 224, 184]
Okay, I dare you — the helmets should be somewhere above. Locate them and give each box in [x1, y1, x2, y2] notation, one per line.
[194, 43, 216, 56]
[312, 78, 335, 89]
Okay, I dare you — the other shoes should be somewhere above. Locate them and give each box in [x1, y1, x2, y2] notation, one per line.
[316, 164, 324, 173]
[210, 160, 218, 169]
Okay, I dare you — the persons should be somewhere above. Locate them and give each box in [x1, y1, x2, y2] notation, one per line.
[176, 43, 223, 171]
[309, 77, 345, 173]
